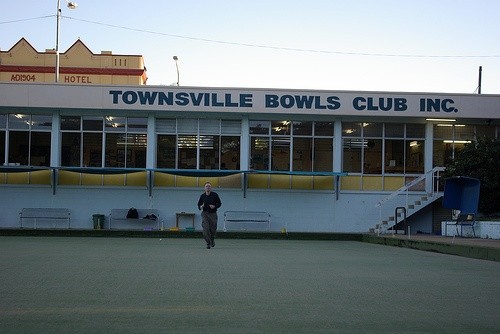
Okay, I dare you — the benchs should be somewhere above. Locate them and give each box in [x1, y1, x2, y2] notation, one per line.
[20, 208, 73, 230]
[108, 209, 160, 230]
[223, 211, 271, 232]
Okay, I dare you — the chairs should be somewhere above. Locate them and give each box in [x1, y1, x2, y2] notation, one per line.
[446, 213, 476, 238]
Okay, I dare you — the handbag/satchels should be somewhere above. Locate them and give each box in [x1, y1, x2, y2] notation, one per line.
[127, 208, 138, 218]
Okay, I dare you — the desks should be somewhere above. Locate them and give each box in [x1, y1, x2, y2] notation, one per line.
[175, 213, 195, 229]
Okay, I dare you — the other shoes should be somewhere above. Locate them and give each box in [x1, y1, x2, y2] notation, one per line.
[207, 243, 210, 249]
[210, 240, 215, 247]
[143, 214, 156, 220]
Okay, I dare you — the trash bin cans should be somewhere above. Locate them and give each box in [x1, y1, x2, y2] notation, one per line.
[92, 215, 105, 230]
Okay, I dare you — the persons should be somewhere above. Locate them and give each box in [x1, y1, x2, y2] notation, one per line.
[198, 182, 222, 249]
